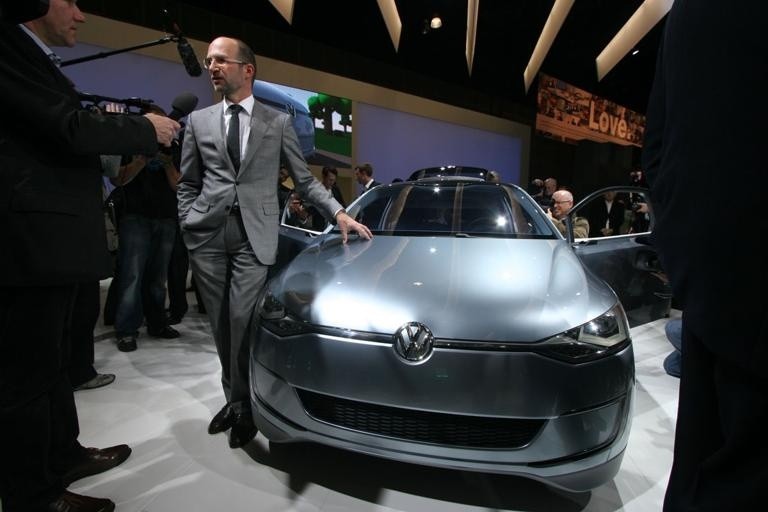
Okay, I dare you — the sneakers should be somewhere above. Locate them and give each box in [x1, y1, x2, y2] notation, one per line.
[162, 308, 185, 326]
[115, 334, 138, 352]
[72, 373, 116, 393]
[148, 326, 181, 339]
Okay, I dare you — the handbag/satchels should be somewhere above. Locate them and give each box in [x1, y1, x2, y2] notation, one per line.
[103, 197, 119, 253]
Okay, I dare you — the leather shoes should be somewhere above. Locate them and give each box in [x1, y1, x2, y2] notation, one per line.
[229, 413, 259, 449]
[208, 402, 239, 435]
[68, 443, 134, 483]
[44, 488, 116, 512]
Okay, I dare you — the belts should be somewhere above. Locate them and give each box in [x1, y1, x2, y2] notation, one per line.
[231, 204, 241, 214]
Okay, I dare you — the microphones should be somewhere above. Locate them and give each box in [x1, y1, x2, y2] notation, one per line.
[178, 41, 201, 77]
[168, 95, 198, 122]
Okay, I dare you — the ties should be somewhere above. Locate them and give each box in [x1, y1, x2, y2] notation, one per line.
[226, 103, 244, 176]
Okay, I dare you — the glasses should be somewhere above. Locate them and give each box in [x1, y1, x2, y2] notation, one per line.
[202, 55, 246, 71]
[555, 201, 568, 203]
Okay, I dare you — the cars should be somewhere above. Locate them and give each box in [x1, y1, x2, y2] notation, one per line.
[250, 82, 321, 165]
[246, 162, 673, 498]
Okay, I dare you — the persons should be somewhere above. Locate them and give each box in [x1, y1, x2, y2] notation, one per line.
[68, 152, 117, 391]
[2, 0, 184, 512]
[633, 0, 768, 511]
[175, 35, 371, 454]
